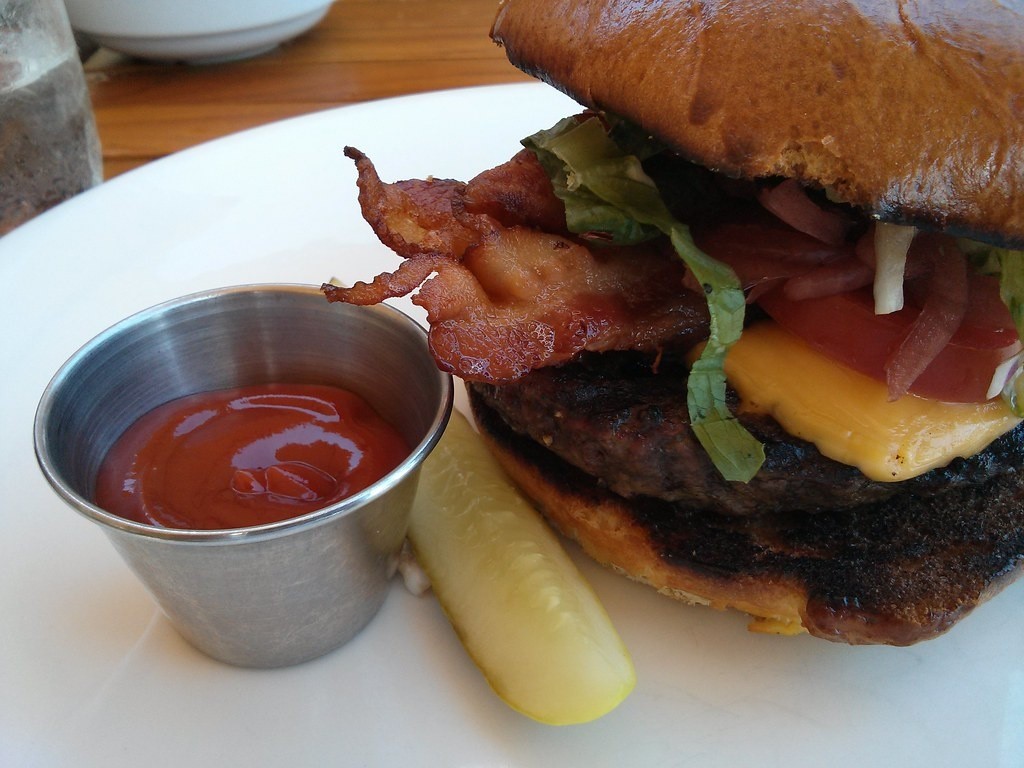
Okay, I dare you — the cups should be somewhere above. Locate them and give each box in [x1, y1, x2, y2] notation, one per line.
[0, 0, 105, 234]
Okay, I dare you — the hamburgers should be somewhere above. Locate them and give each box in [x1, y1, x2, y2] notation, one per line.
[315, 0, 1024, 646]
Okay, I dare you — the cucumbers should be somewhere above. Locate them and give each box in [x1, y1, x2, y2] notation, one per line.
[406, 401, 637, 728]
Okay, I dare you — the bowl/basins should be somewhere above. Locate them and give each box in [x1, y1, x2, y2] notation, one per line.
[64, 0, 337, 66]
[33, 280, 455, 669]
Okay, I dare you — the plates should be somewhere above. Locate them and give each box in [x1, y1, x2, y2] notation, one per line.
[0, 80, 1024, 768]
